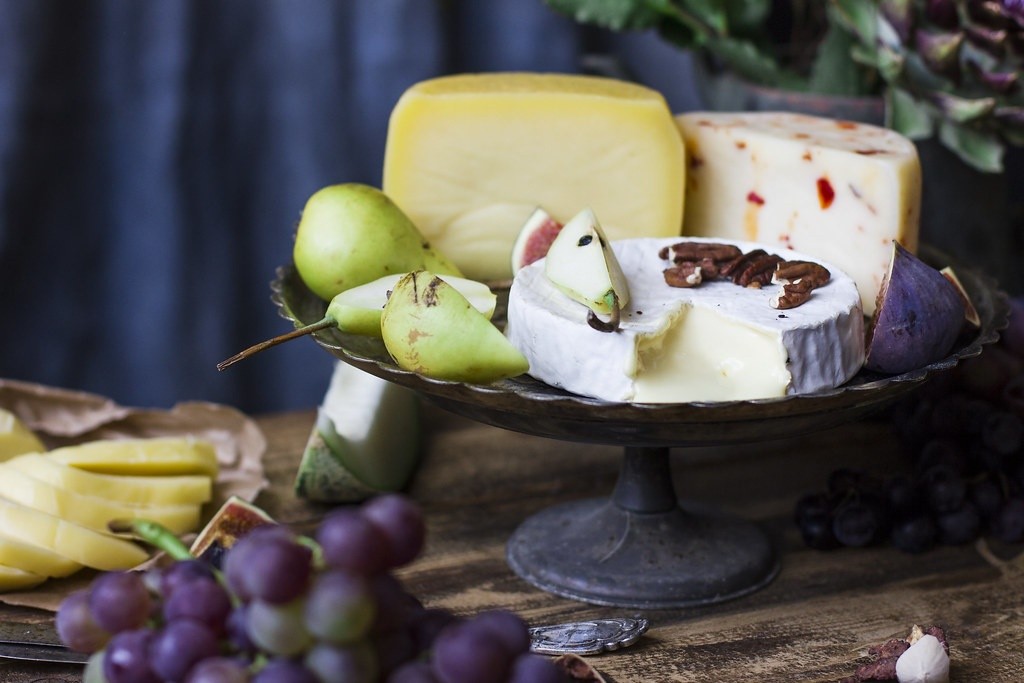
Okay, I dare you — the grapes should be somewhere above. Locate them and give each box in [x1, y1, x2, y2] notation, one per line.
[53, 494, 560, 683]
[796, 295, 1024, 558]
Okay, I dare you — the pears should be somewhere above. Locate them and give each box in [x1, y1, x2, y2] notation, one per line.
[217, 181, 529, 382]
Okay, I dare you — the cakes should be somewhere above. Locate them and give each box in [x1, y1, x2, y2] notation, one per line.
[506, 207, 866, 407]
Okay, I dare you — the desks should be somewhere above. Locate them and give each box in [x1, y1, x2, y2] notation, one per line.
[0, 409, 1023, 683]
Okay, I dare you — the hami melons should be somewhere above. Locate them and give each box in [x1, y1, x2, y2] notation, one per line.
[292, 360, 425, 501]
